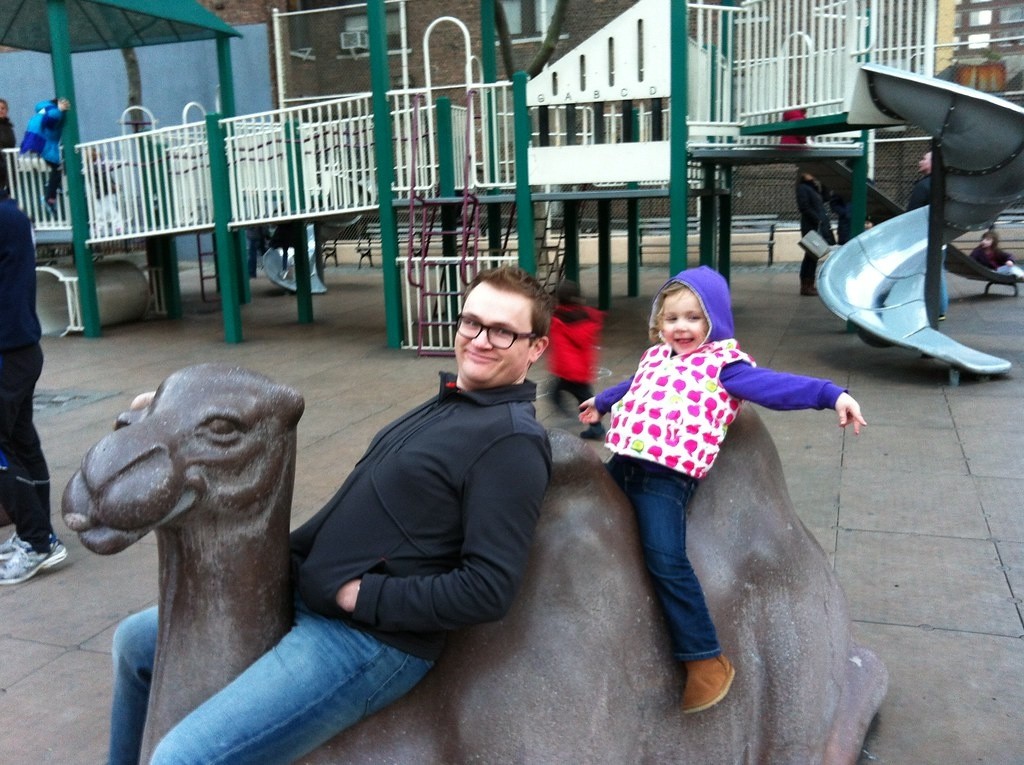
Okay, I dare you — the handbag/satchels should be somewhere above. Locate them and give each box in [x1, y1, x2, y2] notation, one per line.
[797, 230, 829, 258]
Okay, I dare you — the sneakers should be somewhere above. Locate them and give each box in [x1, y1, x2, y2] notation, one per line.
[1, 532, 68, 587]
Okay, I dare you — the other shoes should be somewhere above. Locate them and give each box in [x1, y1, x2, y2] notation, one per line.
[679, 652, 737, 714]
[581, 425, 605, 440]
[937, 315, 946, 321]
[40, 195, 57, 216]
[800, 283, 819, 297]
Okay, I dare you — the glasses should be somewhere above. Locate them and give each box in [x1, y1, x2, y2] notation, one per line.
[455, 313, 533, 350]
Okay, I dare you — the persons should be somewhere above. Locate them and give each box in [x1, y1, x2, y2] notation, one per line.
[109, 269, 559, 765]
[778, 99, 820, 152]
[19, 92, 76, 210]
[269, 219, 301, 271]
[969, 222, 1024, 283]
[581, 264, 869, 714]
[0, 148, 69, 589]
[247, 220, 268, 278]
[795, 166, 837, 297]
[904, 149, 931, 212]
[0, 97, 16, 202]
[545, 278, 608, 443]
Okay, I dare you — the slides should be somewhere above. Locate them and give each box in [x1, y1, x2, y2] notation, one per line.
[817, 65, 1024, 385]
